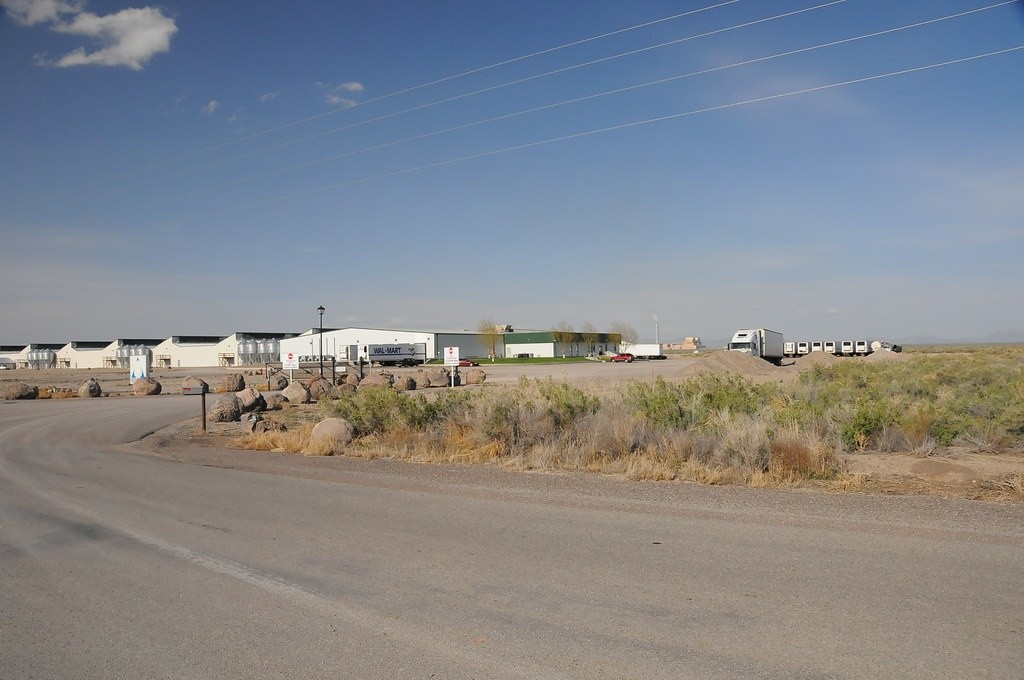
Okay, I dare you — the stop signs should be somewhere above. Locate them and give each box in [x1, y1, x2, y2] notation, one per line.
[289, 353, 293, 359]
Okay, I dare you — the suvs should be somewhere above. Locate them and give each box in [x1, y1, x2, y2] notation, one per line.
[610, 352, 634, 363]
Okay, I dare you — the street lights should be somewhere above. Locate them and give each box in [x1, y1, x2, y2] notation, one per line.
[317, 305, 326, 380]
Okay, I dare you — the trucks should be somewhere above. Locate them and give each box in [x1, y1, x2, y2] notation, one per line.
[783, 340, 900, 357]
[727, 328, 785, 368]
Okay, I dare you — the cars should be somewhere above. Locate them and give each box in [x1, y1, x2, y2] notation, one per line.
[459, 358, 480, 367]
